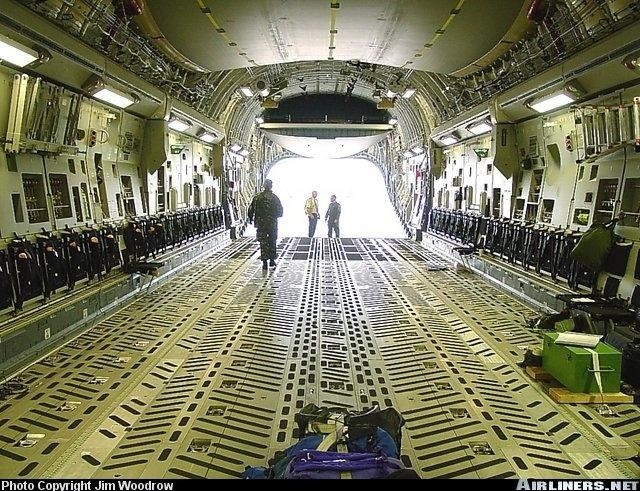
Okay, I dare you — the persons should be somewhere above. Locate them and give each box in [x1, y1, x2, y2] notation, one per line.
[325, 195, 341, 238]
[248, 179, 283, 270]
[304, 191, 320, 238]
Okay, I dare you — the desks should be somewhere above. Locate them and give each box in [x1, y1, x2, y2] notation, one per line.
[557, 294, 632, 338]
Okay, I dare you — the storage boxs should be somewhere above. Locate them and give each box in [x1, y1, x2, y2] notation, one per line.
[542, 333, 622, 393]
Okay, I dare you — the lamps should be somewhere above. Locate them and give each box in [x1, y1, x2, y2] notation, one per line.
[468, 121, 492, 135]
[0, 34, 38, 67]
[530, 90, 577, 113]
[256, 80, 271, 96]
[402, 88, 415, 98]
[441, 135, 459, 145]
[200, 132, 215, 142]
[90, 85, 134, 108]
[168, 119, 190, 132]
[241, 82, 254, 96]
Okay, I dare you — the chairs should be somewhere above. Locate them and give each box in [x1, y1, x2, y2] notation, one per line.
[0, 205, 223, 316]
[431, 208, 597, 289]
